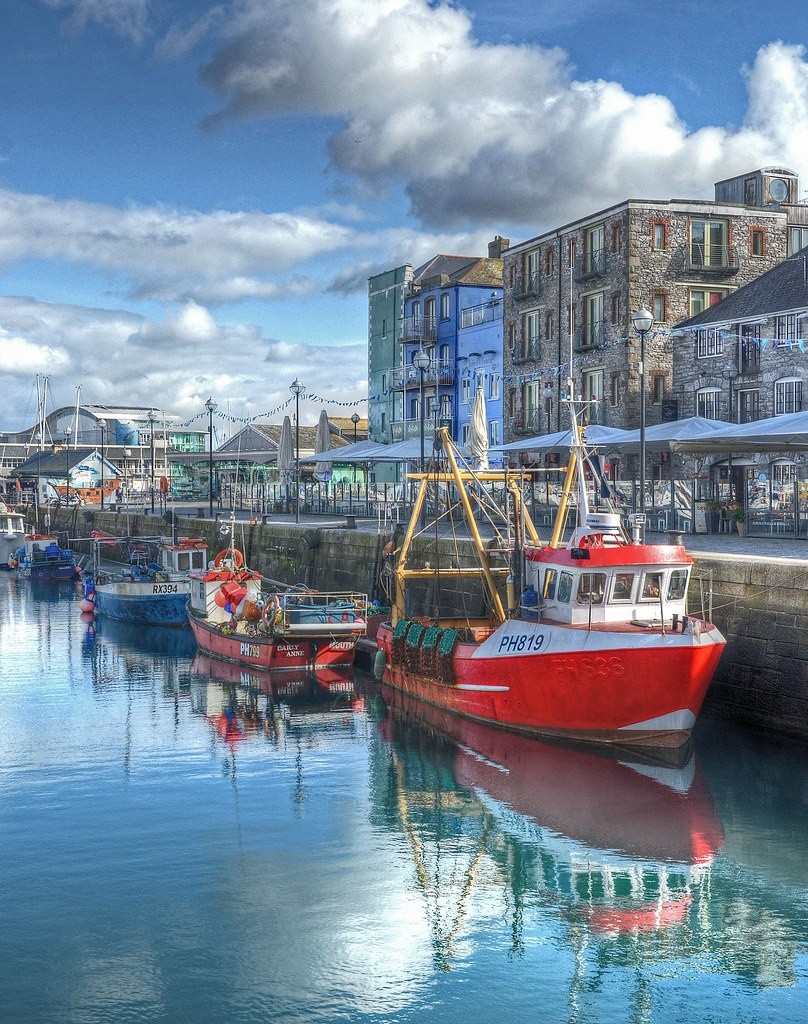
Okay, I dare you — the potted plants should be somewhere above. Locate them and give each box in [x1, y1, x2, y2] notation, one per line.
[705, 502, 721, 535]
[732, 506, 745, 537]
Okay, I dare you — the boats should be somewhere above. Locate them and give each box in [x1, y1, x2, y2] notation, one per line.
[189, 651, 363, 747]
[14, 535, 76, 584]
[376, 237, 727, 745]
[85, 507, 208, 626]
[185, 434, 370, 671]
[378, 684, 726, 1024]
[0, 502, 35, 566]
[87, 610, 199, 665]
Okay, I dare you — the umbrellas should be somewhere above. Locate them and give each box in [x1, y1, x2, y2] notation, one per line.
[311, 410, 332, 505]
[690, 410, 808, 444]
[468, 386, 489, 519]
[588, 417, 739, 531]
[482, 425, 629, 505]
[276, 416, 294, 510]
[345, 438, 471, 474]
[298, 440, 387, 515]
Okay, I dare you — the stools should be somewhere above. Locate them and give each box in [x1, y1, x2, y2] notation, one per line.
[543, 516, 785, 535]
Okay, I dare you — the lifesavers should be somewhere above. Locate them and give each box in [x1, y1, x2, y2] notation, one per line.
[300, 530, 319, 550]
[179, 539, 204, 543]
[215, 548, 243, 569]
[262, 595, 279, 625]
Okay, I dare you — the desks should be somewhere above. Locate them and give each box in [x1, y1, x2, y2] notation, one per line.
[551, 507, 593, 525]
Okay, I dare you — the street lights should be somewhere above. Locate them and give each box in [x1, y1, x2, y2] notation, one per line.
[97, 417, 107, 512]
[204, 396, 218, 517]
[630, 304, 654, 544]
[412, 345, 431, 534]
[148, 408, 158, 513]
[289, 378, 306, 524]
[34, 432, 42, 505]
[63, 425, 71, 508]
[351, 411, 360, 483]
